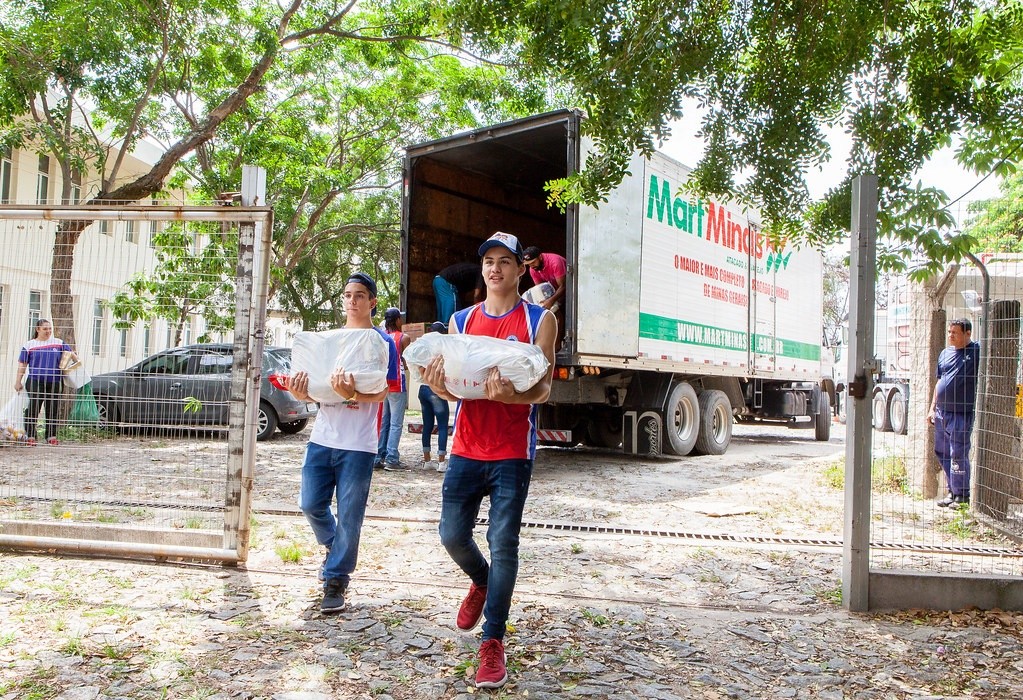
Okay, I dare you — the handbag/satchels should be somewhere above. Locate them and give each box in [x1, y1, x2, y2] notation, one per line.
[71, 383, 99, 421]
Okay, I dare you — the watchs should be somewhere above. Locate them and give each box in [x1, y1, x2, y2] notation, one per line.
[346, 393, 358, 404]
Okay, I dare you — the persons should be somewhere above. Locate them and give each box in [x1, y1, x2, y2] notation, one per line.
[286, 273, 398, 613]
[13, 319, 78, 446]
[928, 318, 981, 511]
[420, 232, 557, 689]
[523, 245, 567, 354]
[433, 262, 485, 324]
[373, 308, 410, 471]
[417, 321, 454, 473]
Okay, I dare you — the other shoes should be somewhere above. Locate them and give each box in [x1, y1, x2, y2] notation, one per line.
[28, 437, 36, 446]
[937, 493, 955, 506]
[949, 496, 969, 509]
[48, 439, 60, 445]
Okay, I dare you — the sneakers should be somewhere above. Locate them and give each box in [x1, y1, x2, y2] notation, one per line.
[456, 582, 489, 632]
[372, 462, 386, 471]
[319, 544, 333, 580]
[422, 460, 439, 470]
[437, 461, 447, 473]
[385, 461, 411, 472]
[320, 578, 345, 612]
[474, 639, 508, 688]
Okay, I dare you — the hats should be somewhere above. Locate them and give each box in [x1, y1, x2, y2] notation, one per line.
[347, 273, 377, 318]
[479, 232, 524, 265]
[523, 246, 539, 264]
[384, 308, 406, 319]
[430, 322, 447, 333]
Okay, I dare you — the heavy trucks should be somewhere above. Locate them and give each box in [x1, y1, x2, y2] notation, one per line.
[831, 305, 1023, 435]
[390, 104, 840, 462]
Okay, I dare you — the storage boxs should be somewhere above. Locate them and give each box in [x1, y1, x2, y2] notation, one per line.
[401, 322, 448, 340]
[63, 361, 92, 389]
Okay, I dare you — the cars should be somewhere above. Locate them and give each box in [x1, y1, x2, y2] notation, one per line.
[78, 340, 320, 442]
